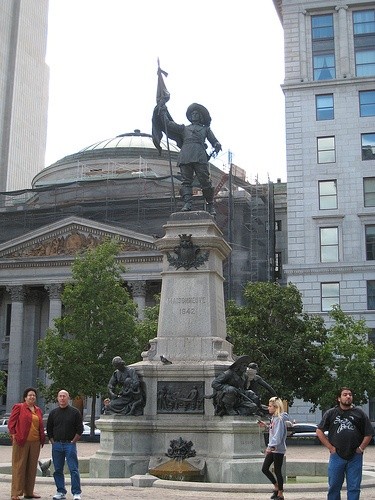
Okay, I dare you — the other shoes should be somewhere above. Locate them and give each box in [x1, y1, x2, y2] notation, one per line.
[274, 495, 284, 500]
[271, 490, 278, 499]
[24, 493, 41, 498]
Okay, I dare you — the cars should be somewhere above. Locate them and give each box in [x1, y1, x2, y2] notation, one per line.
[0, 416, 13, 433]
[291, 422, 329, 437]
[42, 413, 101, 436]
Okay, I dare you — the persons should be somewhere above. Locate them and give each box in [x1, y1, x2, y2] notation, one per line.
[156, 101, 222, 216]
[203, 354, 280, 417]
[46, 390, 85, 500]
[316, 387, 373, 500]
[7, 387, 46, 500]
[106, 356, 147, 416]
[261, 397, 287, 500]
[157, 385, 201, 412]
[101, 398, 111, 415]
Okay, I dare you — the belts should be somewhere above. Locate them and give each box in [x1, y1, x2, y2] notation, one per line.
[54, 439, 71, 442]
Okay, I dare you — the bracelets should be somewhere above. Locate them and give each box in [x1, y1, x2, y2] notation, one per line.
[359, 447, 364, 452]
[71, 441, 76, 444]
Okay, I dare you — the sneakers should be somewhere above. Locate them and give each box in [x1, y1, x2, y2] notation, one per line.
[73, 494, 81, 500]
[53, 493, 66, 500]
